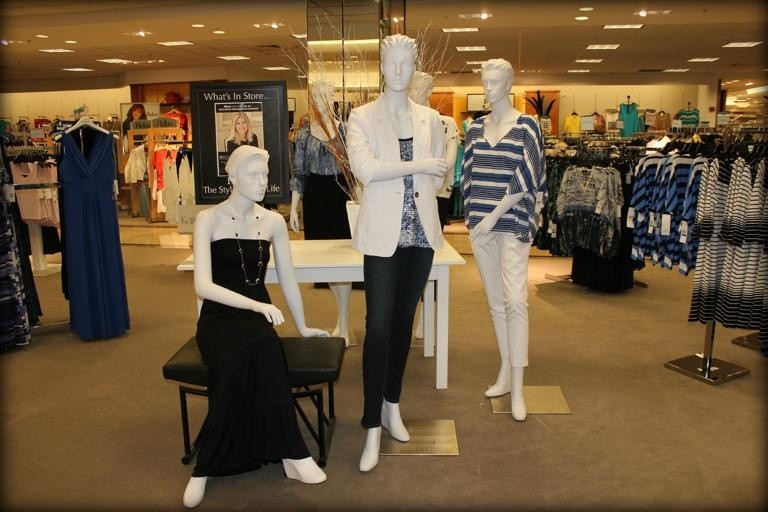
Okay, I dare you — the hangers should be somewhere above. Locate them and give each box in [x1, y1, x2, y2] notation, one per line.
[161, 140, 188, 150]
[4, 103, 120, 168]
[543, 94, 768, 176]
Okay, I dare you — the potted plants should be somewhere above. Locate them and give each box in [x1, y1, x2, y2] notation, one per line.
[281, 10, 467, 240]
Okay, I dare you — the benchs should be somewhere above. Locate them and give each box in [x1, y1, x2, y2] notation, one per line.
[162, 334, 347, 469]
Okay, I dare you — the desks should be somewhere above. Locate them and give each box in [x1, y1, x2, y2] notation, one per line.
[176, 238, 466, 389]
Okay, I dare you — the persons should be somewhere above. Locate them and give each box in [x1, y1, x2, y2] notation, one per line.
[456, 56, 547, 423]
[122, 105, 148, 149]
[407, 68, 459, 231]
[177, 146, 332, 508]
[226, 113, 258, 152]
[344, 29, 452, 475]
[288, 78, 355, 348]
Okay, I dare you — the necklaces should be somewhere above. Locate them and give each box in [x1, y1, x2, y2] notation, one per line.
[228, 195, 264, 286]
